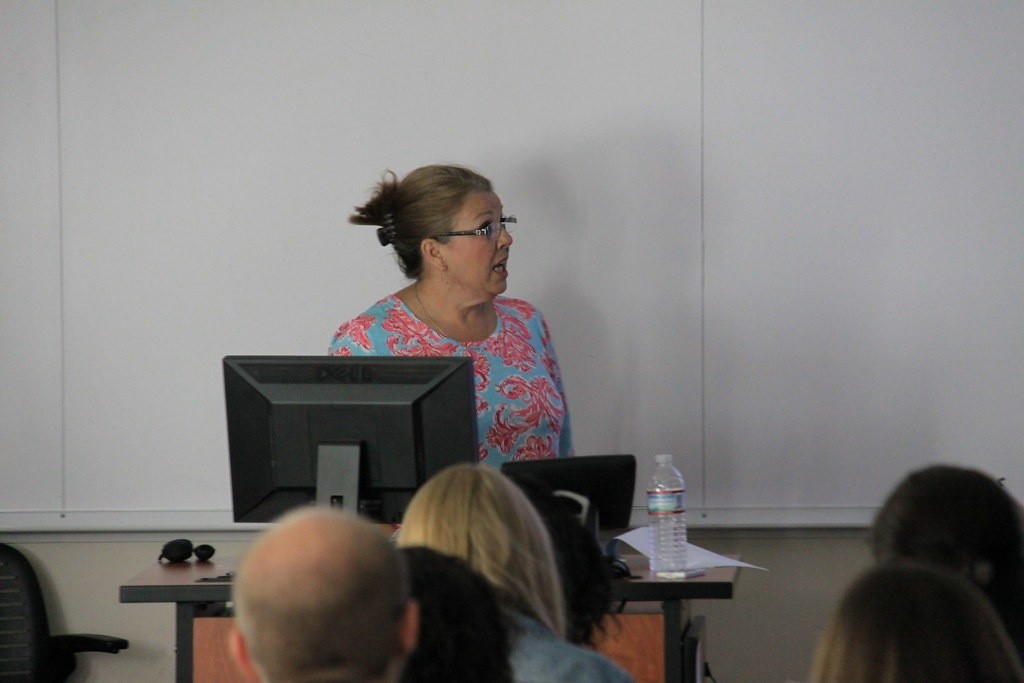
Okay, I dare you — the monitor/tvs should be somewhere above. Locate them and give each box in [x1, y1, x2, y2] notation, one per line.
[224, 355, 480, 526]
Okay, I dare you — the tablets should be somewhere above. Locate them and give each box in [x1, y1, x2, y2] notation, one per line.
[501, 454, 637, 530]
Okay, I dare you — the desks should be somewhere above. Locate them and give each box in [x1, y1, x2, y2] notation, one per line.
[119, 550, 743, 683]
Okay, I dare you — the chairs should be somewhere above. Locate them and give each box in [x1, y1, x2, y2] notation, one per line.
[0, 542, 129, 683]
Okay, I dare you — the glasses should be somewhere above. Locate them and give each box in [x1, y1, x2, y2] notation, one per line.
[440, 214, 518, 239]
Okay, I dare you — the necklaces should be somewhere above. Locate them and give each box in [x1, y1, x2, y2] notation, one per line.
[415, 282, 488, 341]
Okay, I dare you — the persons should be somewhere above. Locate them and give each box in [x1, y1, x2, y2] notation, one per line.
[228, 504, 417, 683]
[809, 563, 1024, 683]
[399, 546, 515, 683]
[326, 164, 575, 473]
[394, 461, 637, 683]
[868, 464, 1024, 658]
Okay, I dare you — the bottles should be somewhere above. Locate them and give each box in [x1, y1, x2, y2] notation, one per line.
[646, 454, 687, 574]
